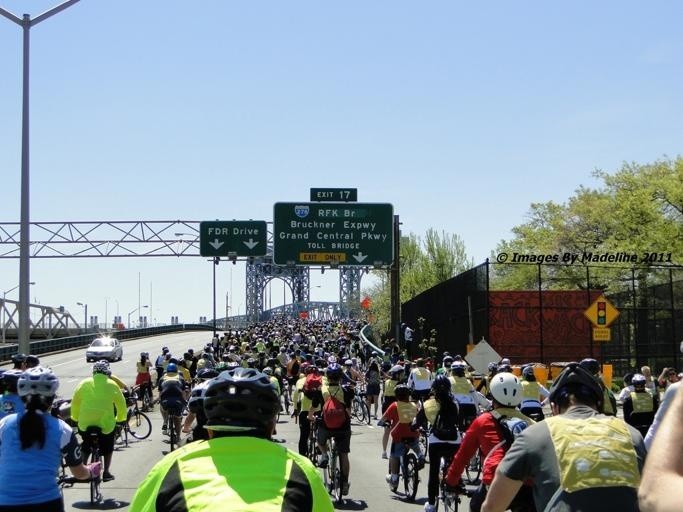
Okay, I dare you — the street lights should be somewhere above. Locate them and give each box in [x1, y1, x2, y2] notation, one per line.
[127, 306, 149, 331]
[2, 282, 36, 344]
[76, 303, 87, 333]
[174, 232, 215, 337]
[303, 284, 321, 290]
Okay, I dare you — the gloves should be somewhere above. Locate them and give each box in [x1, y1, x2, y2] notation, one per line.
[88, 461, 102, 478]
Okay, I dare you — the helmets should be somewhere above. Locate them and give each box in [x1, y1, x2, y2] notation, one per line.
[549, 362, 604, 414]
[300, 355, 353, 375]
[580, 357, 600, 376]
[188, 363, 283, 422]
[140, 352, 149, 358]
[93, 359, 110, 376]
[183, 343, 256, 363]
[431, 375, 451, 392]
[632, 374, 646, 385]
[417, 358, 426, 365]
[162, 347, 177, 372]
[372, 351, 378, 355]
[389, 364, 404, 373]
[443, 352, 466, 370]
[0, 354, 59, 397]
[487, 358, 534, 377]
[394, 384, 411, 395]
[489, 372, 524, 406]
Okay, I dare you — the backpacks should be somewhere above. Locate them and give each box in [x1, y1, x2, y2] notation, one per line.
[456, 394, 478, 432]
[483, 408, 529, 471]
[302, 374, 323, 393]
[324, 388, 346, 428]
[432, 403, 458, 440]
[521, 398, 545, 422]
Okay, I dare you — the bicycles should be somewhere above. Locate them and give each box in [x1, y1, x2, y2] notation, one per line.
[58, 424, 103, 508]
[350, 373, 389, 425]
[377, 398, 481, 512]
[113, 377, 191, 459]
[273, 372, 344, 505]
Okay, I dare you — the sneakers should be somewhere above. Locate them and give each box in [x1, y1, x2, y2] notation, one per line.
[424, 501, 436, 512]
[162, 425, 166, 430]
[343, 483, 349, 496]
[382, 452, 387, 458]
[417, 453, 425, 467]
[319, 453, 329, 468]
[385, 474, 399, 488]
[103, 473, 115, 482]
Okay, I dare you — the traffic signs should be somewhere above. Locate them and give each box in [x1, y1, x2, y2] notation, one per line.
[198, 221, 266, 257]
[272, 202, 395, 268]
[309, 187, 357, 203]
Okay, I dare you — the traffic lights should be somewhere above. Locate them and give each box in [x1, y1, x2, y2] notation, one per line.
[595, 300, 605, 325]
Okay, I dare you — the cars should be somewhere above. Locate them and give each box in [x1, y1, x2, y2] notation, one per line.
[85, 338, 123, 362]
